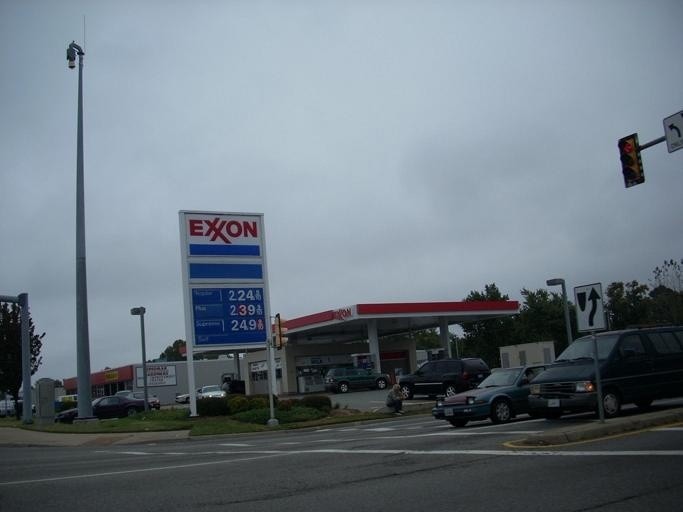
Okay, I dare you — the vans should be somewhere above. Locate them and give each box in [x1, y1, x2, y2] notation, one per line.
[528, 325, 683, 418]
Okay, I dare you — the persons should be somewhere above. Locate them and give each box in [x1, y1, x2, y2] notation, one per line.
[385, 382, 406, 417]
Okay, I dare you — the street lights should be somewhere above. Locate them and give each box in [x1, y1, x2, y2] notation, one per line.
[546, 278, 573, 345]
[67, 40, 96, 421]
[130, 306, 150, 411]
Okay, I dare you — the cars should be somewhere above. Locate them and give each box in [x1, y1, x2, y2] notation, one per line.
[176, 385, 227, 404]
[432, 364, 552, 426]
[399, 357, 490, 400]
[325, 368, 391, 393]
[54, 390, 160, 424]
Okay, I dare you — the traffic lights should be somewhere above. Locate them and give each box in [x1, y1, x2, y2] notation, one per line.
[274, 313, 288, 350]
[618, 132, 645, 188]
